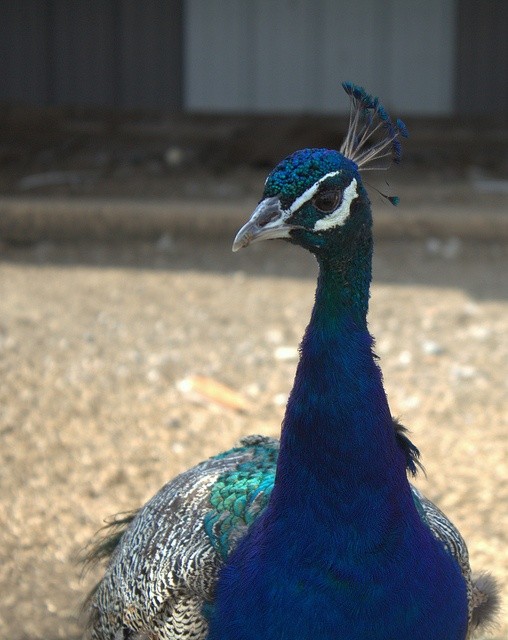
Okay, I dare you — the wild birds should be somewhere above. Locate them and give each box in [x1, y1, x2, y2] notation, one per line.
[78, 81, 502, 640]
